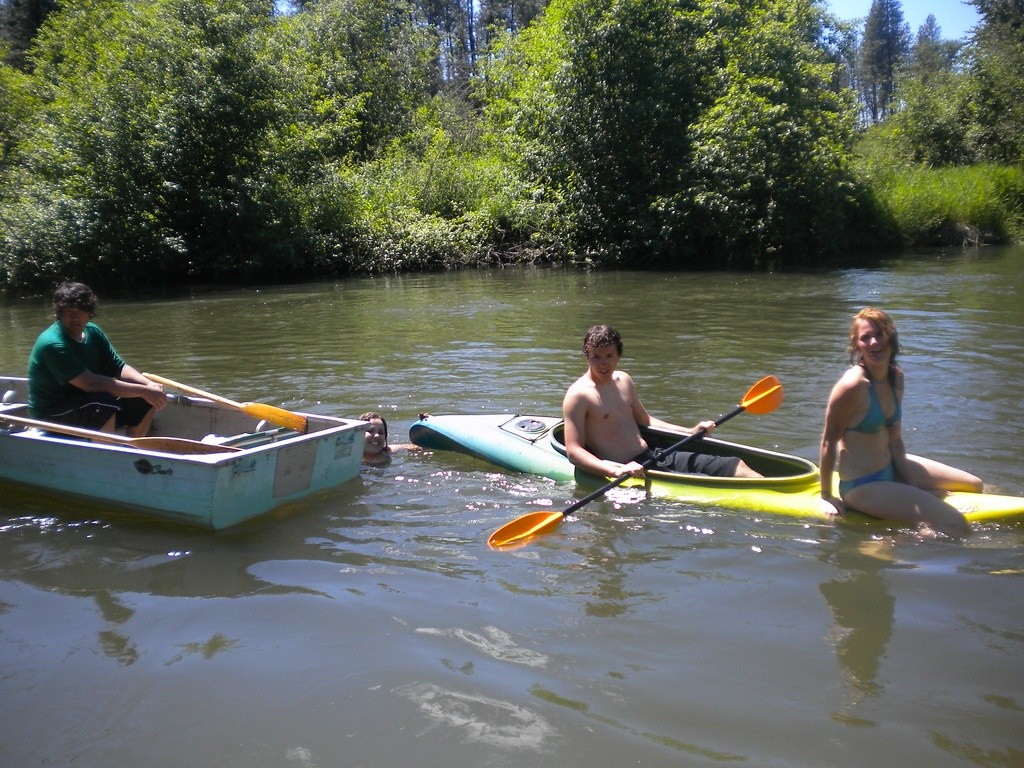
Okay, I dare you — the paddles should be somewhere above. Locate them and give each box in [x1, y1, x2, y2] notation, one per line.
[2, 408, 246, 456]
[488, 374, 785, 556]
[142, 368, 309, 435]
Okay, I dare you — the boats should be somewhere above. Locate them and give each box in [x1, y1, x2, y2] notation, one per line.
[1, 374, 371, 530]
[409, 406, 1023, 538]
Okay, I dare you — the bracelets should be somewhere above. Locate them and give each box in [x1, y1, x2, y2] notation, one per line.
[821, 490, 831, 493]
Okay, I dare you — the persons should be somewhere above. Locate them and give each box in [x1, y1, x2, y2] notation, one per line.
[27, 283, 167, 450]
[820, 307, 982, 539]
[562, 325, 764, 480]
[358, 412, 430, 463]
